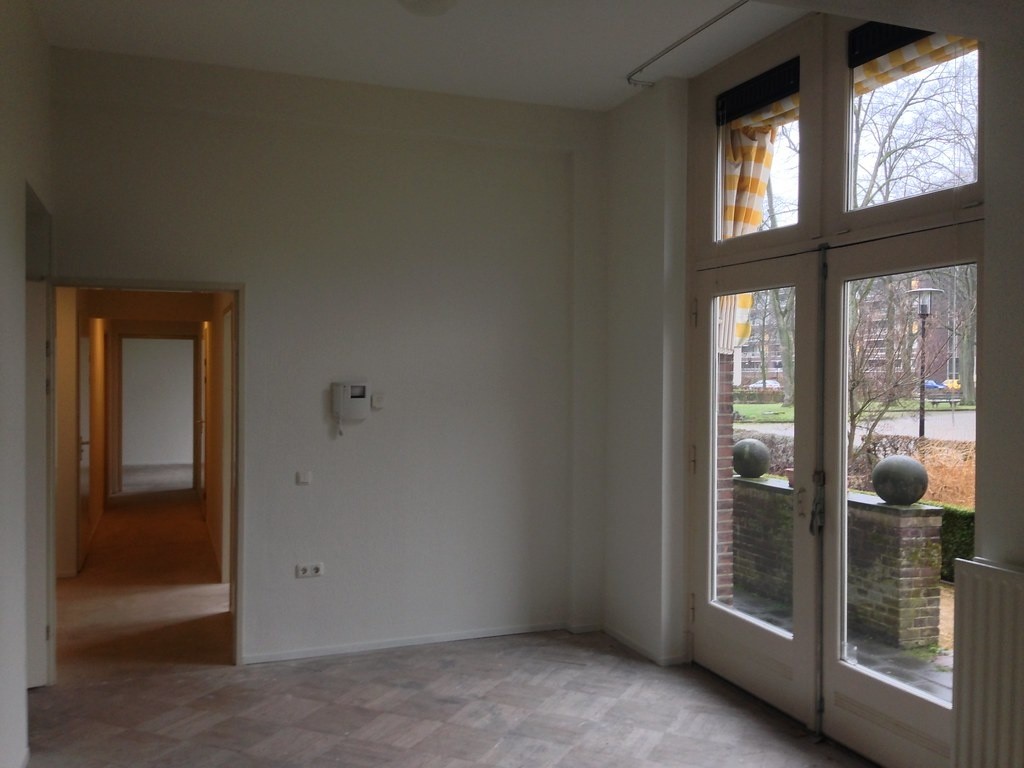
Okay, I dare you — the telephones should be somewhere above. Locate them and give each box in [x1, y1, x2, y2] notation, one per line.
[331, 381, 374, 422]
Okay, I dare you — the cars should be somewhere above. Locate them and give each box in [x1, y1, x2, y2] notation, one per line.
[748, 380, 780, 390]
[924, 380, 946, 389]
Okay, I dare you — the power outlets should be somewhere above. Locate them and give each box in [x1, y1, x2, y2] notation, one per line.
[295, 561, 325, 578]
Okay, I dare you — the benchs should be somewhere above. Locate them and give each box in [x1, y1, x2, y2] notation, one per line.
[927, 399, 962, 408]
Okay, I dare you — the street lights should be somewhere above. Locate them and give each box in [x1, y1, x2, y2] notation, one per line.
[906, 289, 945, 463]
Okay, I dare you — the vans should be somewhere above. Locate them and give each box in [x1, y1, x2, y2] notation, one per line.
[940, 380, 960, 389]
